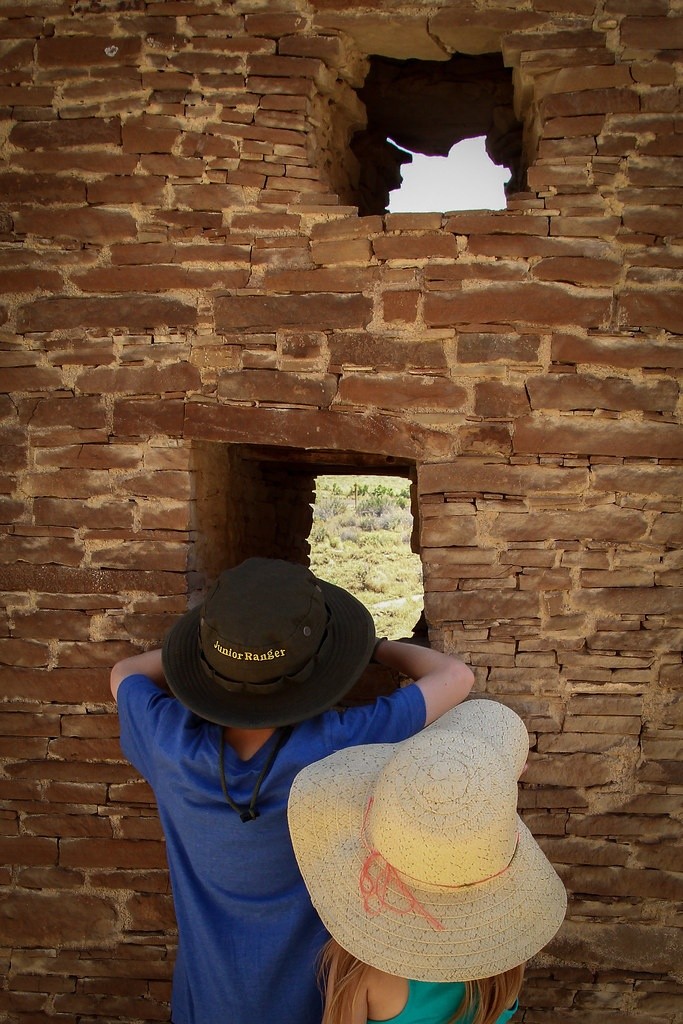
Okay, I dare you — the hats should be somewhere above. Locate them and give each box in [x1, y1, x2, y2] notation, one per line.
[161, 557, 376, 729]
[286, 698, 567, 982]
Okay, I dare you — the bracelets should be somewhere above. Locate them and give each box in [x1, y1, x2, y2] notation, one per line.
[372, 637, 388, 664]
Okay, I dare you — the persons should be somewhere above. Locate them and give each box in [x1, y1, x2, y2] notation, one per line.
[109, 557, 476, 1024]
[286, 699, 568, 1024]
